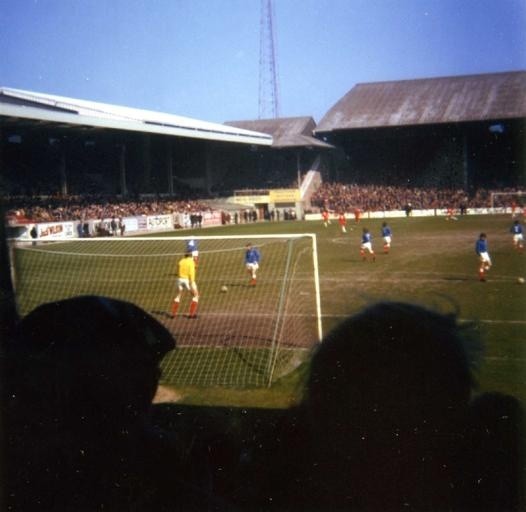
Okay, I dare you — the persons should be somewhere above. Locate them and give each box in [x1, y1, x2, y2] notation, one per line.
[339, 210, 347, 232]
[322, 207, 331, 227]
[475, 232, 492, 282]
[2, 180, 212, 246]
[221, 208, 296, 225]
[511, 221, 525, 250]
[353, 207, 360, 224]
[381, 222, 392, 253]
[171, 241, 200, 319]
[2, 296, 180, 511]
[311, 171, 525, 221]
[263, 301, 525, 511]
[244, 243, 260, 287]
[360, 228, 376, 261]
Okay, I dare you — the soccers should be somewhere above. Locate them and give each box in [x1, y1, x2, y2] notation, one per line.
[221, 286, 227, 292]
[517, 276, 524, 283]
